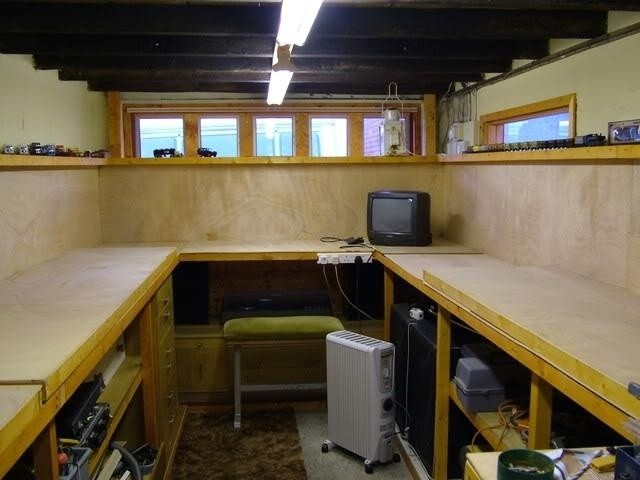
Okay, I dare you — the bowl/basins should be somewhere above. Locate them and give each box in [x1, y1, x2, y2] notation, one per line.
[497, 448, 555, 480]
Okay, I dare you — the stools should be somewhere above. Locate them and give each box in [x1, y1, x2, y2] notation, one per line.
[223, 317, 346, 432]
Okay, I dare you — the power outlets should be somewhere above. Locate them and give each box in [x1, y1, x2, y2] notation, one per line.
[410, 308, 424, 320]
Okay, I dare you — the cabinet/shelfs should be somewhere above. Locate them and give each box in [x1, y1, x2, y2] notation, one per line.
[460, 448, 640, 479]
[144, 276, 190, 454]
[176, 254, 393, 402]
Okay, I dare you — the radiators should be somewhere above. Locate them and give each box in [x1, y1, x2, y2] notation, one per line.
[321, 330, 400, 473]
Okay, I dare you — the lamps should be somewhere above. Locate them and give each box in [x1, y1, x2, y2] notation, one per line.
[261, 1, 324, 105]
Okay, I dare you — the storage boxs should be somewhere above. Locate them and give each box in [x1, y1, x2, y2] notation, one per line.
[454, 359, 527, 411]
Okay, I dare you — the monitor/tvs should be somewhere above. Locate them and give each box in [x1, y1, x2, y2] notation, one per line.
[366, 190, 432, 247]
